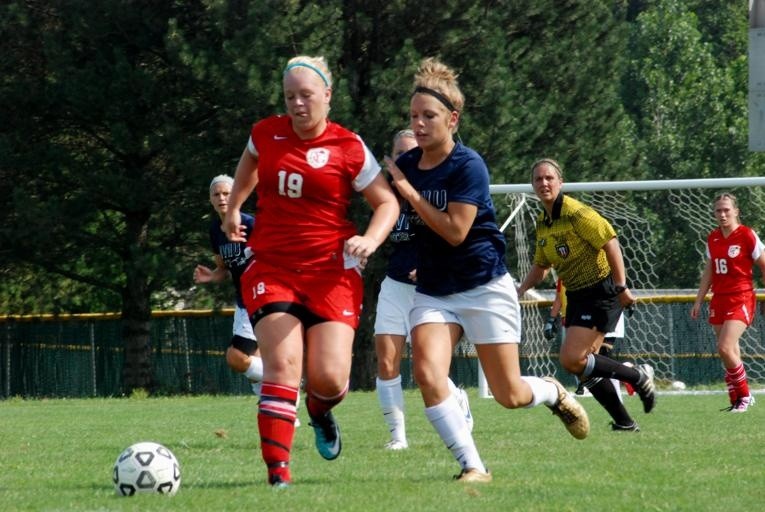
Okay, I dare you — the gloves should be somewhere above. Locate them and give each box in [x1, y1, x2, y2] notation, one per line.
[543, 316, 559, 342]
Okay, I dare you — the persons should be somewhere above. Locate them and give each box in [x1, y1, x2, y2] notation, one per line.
[191, 174, 301, 429]
[382, 54, 590, 484]
[544, 274, 637, 408]
[690, 192, 765, 413]
[220, 54, 402, 489]
[376, 127, 474, 453]
[515, 159, 656, 433]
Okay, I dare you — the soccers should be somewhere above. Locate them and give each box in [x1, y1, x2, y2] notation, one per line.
[673, 381, 686, 391]
[113, 443, 181, 498]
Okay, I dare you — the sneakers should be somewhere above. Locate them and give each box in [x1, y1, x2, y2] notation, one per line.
[453, 387, 474, 434]
[619, 361, 636, 397]
[450, 466, 492, 486]
[632, 363, 658, 414]
[382, 438, 409, 451]
[541, 375, 590, 444]
[605, 419, 642, 436]
[719, 393, 757, 416]
[304, 396, 344, 461]
